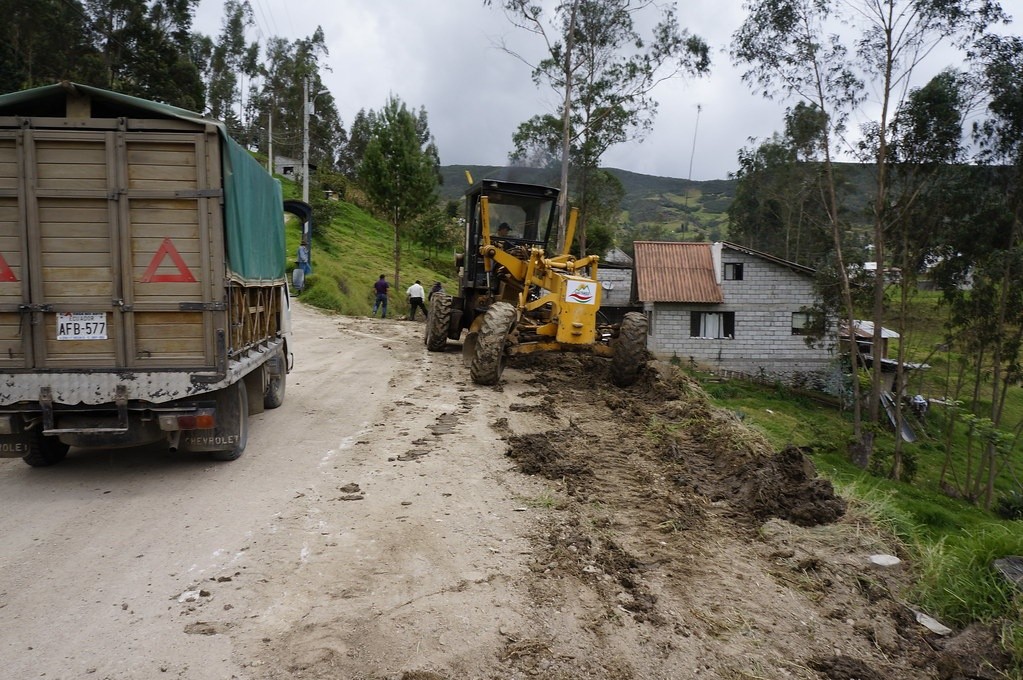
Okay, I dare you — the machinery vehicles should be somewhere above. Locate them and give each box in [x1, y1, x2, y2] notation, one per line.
[425, 178, 650, 389]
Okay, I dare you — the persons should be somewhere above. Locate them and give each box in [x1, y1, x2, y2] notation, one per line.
[489, 222, 512, 246]
[428, 282, 443, 301]
[406, 280, 428, 321]
[371, 274, 389, 318]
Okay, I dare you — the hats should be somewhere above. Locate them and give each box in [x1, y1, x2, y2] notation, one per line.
[497, 222, 512, 231]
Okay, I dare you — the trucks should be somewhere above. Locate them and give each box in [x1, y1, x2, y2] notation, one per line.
[0, 78, 311, 470]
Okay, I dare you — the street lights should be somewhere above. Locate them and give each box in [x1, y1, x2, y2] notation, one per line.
[302, 76, 331, 202]
